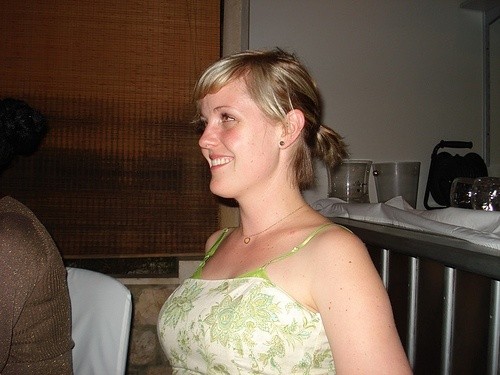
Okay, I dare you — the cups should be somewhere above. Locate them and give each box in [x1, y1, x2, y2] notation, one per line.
[327, 159, 372, 204]
[373, 161, 421, 208]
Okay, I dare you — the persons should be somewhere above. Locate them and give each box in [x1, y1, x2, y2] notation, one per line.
[156, 46, 416, 375]
[0, 97, 75, 375]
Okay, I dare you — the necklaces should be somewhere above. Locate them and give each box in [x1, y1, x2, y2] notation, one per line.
[240, 202, 306, 244]
